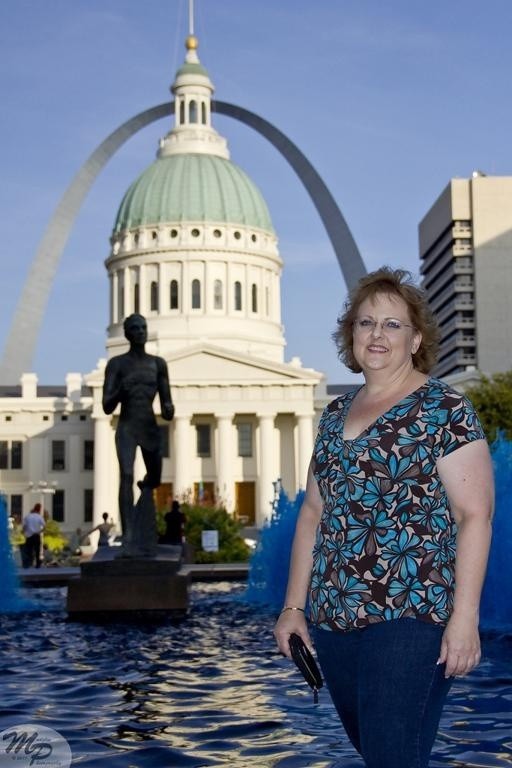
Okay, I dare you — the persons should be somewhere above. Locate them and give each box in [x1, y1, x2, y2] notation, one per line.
[8, 512, 18, 530]
[164, 501, 187, 542]
[101, 313, 173, 541]
[88, 512, 114, 549]
[68, 525, 84, 551]
[21, 502, 46, 568]
[271, 264, 497, 767]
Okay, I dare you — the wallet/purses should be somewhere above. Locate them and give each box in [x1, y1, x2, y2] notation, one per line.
[289, 634, 324, 691]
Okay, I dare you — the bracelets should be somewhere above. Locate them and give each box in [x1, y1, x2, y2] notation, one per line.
[280, 607, 305, 613]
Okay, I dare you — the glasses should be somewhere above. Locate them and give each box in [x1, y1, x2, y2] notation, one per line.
[352, 318, 417, 331]
